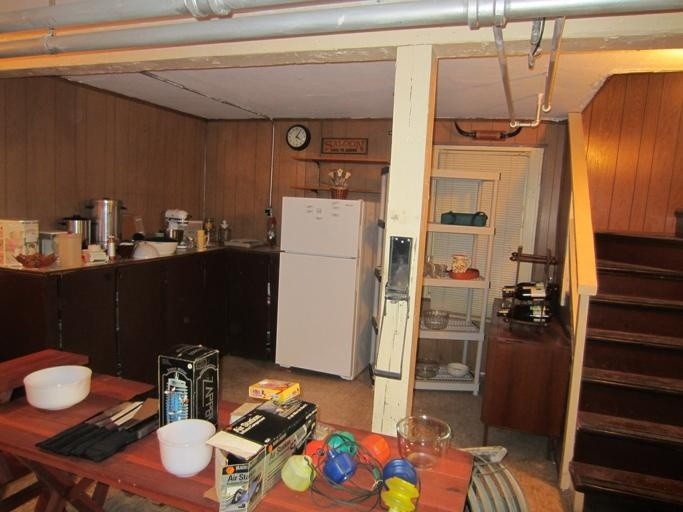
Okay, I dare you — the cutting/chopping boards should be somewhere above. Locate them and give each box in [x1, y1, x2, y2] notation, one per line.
[225, 239, 262, 248]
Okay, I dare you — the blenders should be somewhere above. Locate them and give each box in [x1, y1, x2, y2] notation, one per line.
[164, 208, 189, 248]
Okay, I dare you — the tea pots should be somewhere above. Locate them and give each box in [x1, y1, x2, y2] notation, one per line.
[452, 255, 472, 273]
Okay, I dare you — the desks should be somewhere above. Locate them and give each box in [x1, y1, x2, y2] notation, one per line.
[1, 348, 474, 512]
[480, 297, 574, 459]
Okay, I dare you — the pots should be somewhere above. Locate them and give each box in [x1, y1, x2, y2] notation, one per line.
[86, 197, 127, 248]
[58, 214, 93, 248]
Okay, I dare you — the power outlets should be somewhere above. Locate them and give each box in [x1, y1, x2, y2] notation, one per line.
[265, 208, 272, 217]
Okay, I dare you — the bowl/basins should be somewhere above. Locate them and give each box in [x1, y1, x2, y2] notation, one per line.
[447, 362, 470, 378]
[156, 419, 216, 478]
[396, 414, 451, 470]
[416, 355, 440, 378]
[23, 365, 93, 410]
[425, 309, 449, 330]
[149, 240, 179, 257]
[131, 241, 160, 260]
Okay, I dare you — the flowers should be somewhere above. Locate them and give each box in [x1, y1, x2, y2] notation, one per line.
[328, 168, 351, 187]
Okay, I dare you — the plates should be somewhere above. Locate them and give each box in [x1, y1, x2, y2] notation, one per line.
[11, 252, 59, 268]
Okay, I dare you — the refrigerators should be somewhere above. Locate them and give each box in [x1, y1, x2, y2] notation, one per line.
[274, 196, 381, 380]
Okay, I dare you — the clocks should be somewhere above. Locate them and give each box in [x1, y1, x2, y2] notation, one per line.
[285, 124, 310, 150]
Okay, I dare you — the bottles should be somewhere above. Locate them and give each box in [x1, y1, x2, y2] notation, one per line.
[218, 219, 232, 244]
[497, 281, 559, 323]
[107, 234, 116, 260]
[197, 229, 205, 249]
[203, 217, 216, 241]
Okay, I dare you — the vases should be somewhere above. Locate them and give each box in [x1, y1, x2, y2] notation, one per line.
[330, 187, 348, 199]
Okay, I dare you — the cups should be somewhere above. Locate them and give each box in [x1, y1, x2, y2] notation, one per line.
[425, 262, 447, 278]
[50, 232, 82, 267]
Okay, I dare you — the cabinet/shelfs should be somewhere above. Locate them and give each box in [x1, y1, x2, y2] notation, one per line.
[166, 254, 226, 356]
[0, 259, 164, 385]
[226, 251, 279, 359]
[414, 170, 502, 396]
